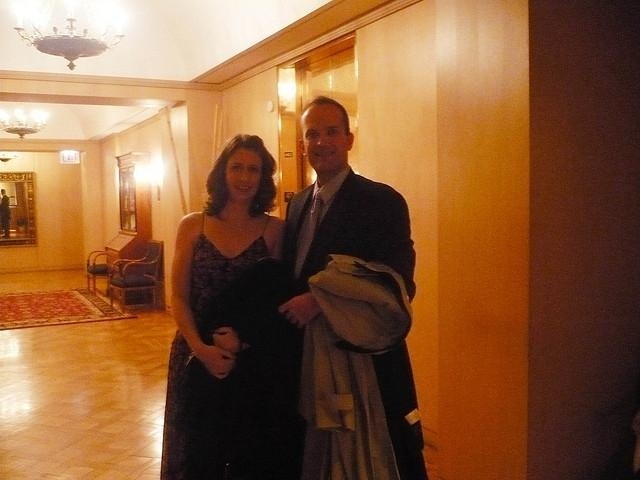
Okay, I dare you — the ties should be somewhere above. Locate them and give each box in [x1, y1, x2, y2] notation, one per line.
[295, 195, 324, 278]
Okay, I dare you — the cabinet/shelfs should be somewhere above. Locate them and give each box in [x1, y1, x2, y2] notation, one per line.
[105, 151, 151, 269]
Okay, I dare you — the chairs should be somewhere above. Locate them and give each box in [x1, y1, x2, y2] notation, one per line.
[87, 238, 165, 313]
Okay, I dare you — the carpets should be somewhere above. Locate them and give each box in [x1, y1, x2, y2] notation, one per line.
[1, 288, 136, 329]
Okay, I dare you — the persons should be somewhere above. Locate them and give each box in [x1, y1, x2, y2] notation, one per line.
[0, 189, 10, 238]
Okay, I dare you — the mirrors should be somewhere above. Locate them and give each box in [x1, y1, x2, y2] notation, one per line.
[0, 173, 39, 248]
